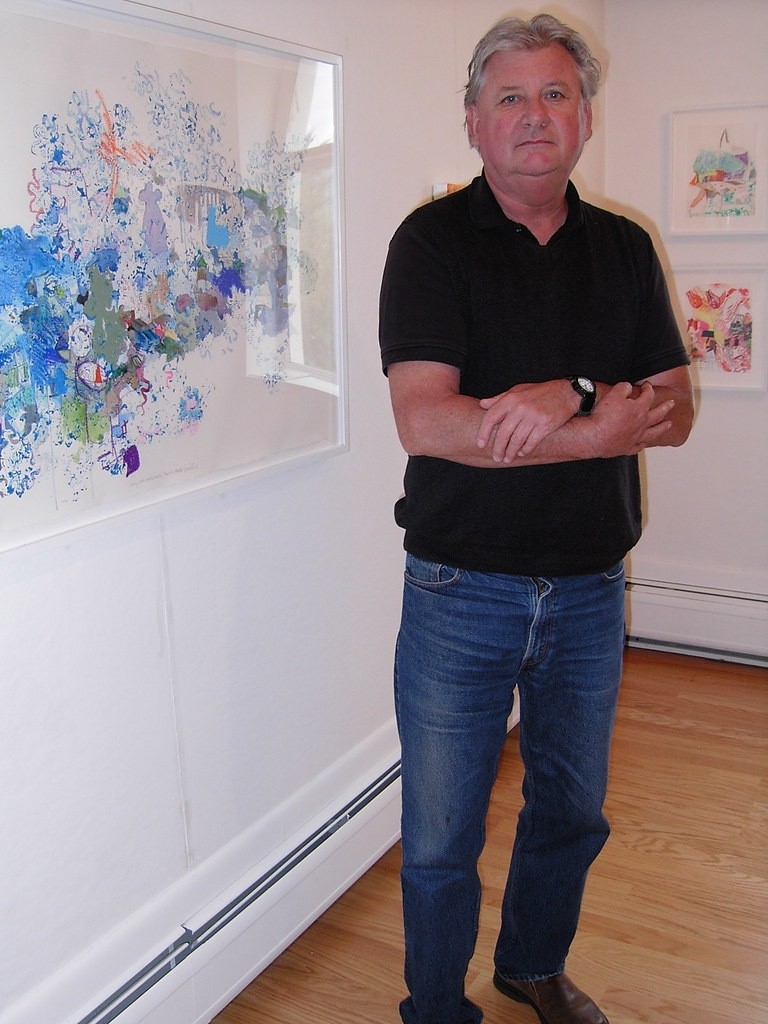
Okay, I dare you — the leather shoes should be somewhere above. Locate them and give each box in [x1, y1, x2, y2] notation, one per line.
[493, 968, 611, 1024]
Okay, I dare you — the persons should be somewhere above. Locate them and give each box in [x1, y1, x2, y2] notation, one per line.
[381, 6, 695, 1024]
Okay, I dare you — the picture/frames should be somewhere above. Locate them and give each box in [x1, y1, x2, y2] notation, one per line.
[661, 263, 767, 393]
[661, 98, 768, 244]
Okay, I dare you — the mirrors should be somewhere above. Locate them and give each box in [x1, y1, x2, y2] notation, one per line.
[0, 0, 352, 565]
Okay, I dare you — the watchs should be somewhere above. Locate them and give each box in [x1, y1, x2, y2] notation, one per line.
[568, 374, 597, 418]
